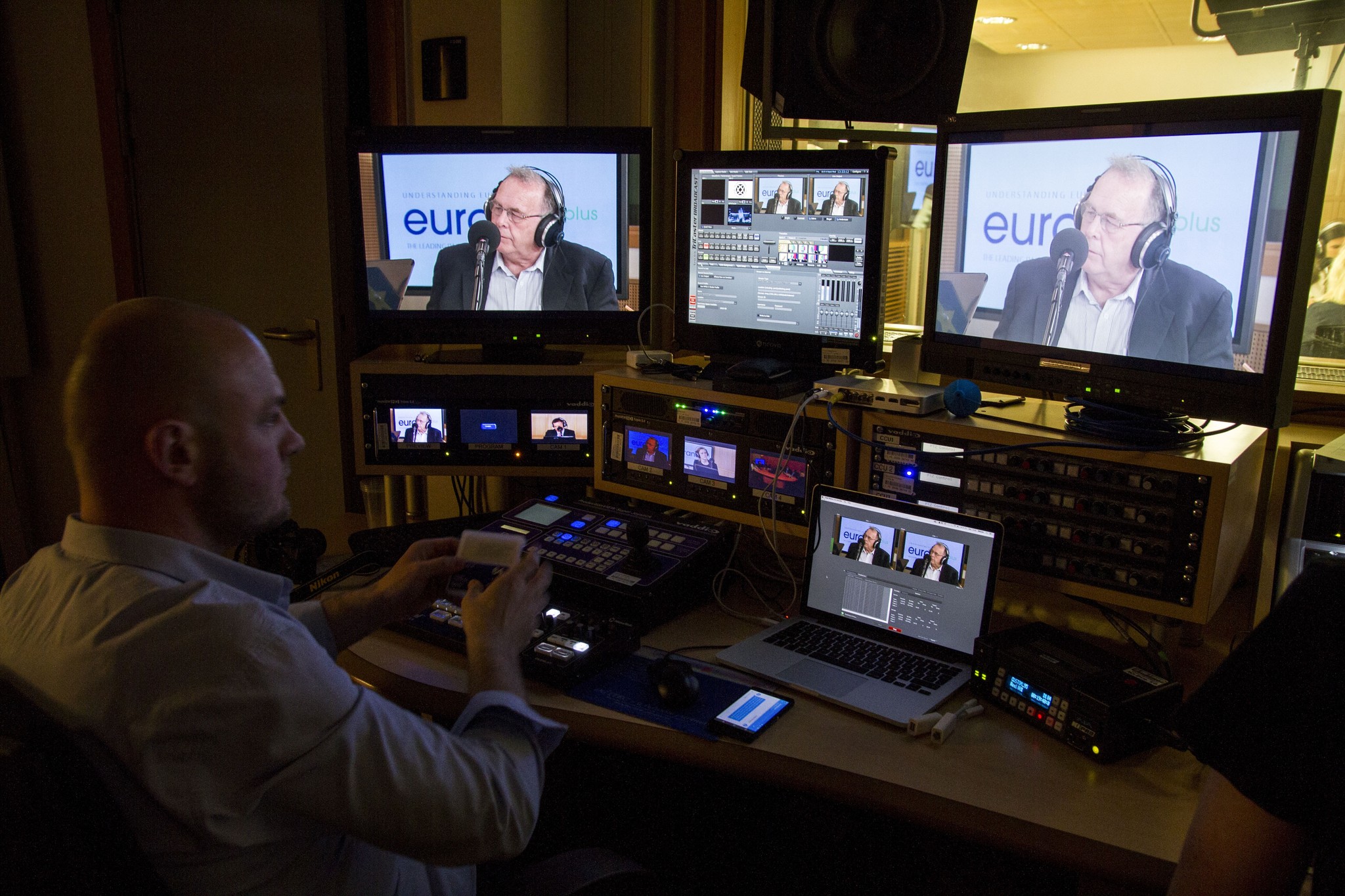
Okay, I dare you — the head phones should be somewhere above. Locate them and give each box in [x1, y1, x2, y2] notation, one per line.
[646, 437, 659, 452]
[833, 181, 850, 201]
[483, 167, 566, 248]
[695, 446, 708, 458]
[415, 412, 432, 429]
[1073, 155, 1176, 271]
[1315, 222, 1345, 258]
[929, 542, 949, 565]
[863, 527, 881, 548]
[777, 181, 792, 200]
[552, 418, 567, 427]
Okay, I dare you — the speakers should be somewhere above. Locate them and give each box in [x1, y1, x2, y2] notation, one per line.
[741, 0, 977, 125]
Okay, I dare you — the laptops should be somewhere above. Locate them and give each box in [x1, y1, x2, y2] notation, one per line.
[716, 483, 1004, 728]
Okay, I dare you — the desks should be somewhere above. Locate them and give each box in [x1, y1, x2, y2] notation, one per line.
[222, 510, 1313, 895]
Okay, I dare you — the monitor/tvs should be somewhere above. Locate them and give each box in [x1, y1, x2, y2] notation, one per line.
[913, 90, 1341, 424]
[674, 149, 896, 376]
[331, 128, 658, 367]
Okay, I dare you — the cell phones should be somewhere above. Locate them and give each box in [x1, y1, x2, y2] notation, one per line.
[707, 687, 794, 743]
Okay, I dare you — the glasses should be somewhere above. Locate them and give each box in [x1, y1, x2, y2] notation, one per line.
[778, 188, 788, 193]
[645, 442, 655, 446]
[418, 419, 427, 423]
[930, 549, 943, 557]
[489, 199, 546, 224]
[834, 189, 845, 194]
[863, 533, 876, 541]
[1080, 201, 1146, 232]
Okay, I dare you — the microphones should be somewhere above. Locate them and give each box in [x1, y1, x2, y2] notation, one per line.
[411, 423, 418, 443]
[828, 194, 836, 216]
[642, 445, 647, 461]
[467, 220, 501, 311]
[557, 427, 563, 436]
[855, 538, 865, 561]
[1040, 228, 1089, 347]
[920, 554, 931, 578]
[709, 458, 714, 468]
[773, 193, 780, 214]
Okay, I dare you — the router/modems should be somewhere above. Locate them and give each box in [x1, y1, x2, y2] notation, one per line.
[813, 374, 948, 416]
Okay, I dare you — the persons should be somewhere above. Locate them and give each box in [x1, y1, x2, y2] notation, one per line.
[766, 180, 803, 215]
[404, 410, 443, 442]
[635, 438, 669, 465]
[816, 181, 861, 218]
[0, 292, 568, 894]
[421, 161, 618, 310]
[691, 448, 721, 476]
[843, 527, 891, 567]
[992, 154, 1237, 374]
[910, 543, 962, 586]
[544, 419, 578, 441]
